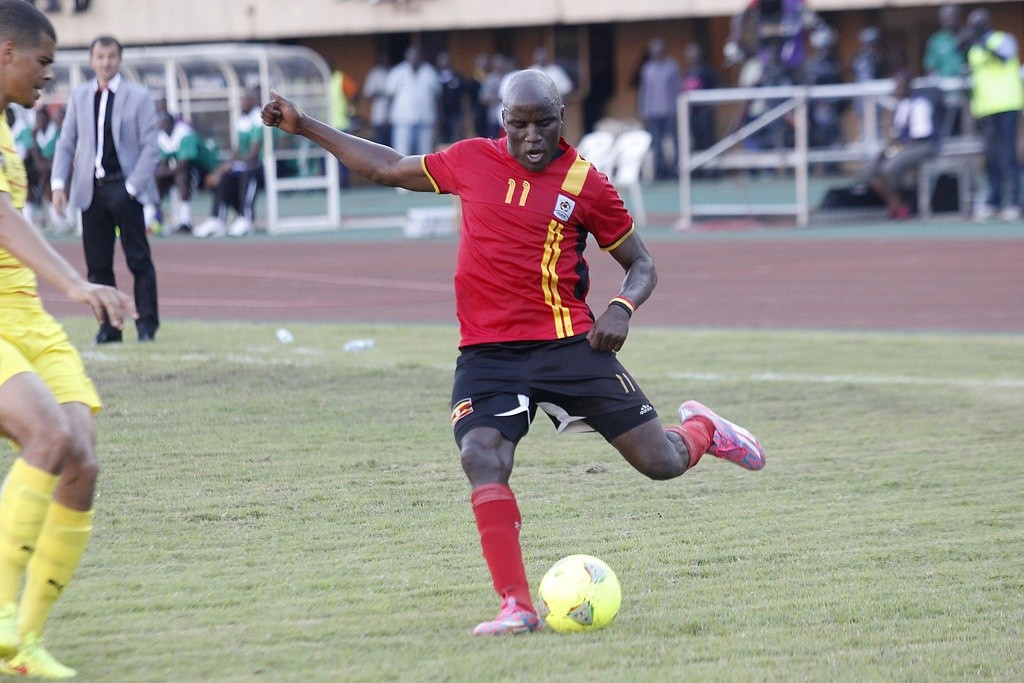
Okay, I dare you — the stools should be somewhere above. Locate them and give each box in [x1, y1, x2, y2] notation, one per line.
[918, 156, 969, 220]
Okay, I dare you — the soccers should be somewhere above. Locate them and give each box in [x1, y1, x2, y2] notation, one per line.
[537, 554, 621, 634]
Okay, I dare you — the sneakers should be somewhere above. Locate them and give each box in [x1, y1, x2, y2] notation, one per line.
[0, 599, 20, 659]
[473, 598, 543, 635]
[0, 640, 78, 680]
[679, 401, 767, 470]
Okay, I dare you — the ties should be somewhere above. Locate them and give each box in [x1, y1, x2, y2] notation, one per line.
[94, 88, 111, 178]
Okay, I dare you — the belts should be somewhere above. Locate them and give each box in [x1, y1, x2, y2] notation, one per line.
[94, 171, 125, 182]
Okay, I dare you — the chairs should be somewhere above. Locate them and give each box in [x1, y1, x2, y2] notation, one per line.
[599, 129, 652, 225]
[574, 131, 614, 176]
[223, 130, 299, 226]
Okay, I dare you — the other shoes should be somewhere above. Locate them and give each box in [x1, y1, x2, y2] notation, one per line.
[999, 206, 1021, 221]
[94, 329, 123, 344]
[177, 212, 193, 234]
[884, 205, 911, 219]
[229, 215, 257, 237]
[138, 330, 155, 341]
[972, 205, 999, 225]
[142, 203, 162, 233]
[195, 216, 227, 238]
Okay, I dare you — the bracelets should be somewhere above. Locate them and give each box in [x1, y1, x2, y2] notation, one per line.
[607, 296, 636, 319]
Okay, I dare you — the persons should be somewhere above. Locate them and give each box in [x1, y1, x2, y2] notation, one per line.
[0, 0, 139, 679]
[362, 43, 574, 193]
[6, 106, 61, 234]
[741, 24, 883, 176]
[50, 35, 163, 345]
[873, 67, 938, 219]
[921, 3, 967, 136]
[192, 89, 266, 239]
[637, 38, 722, 181]
[320, 54, 359, 190]
[260, 68, 767, 637]
[957, 7, 1024, 221]
[154, 112, 217, 231]
